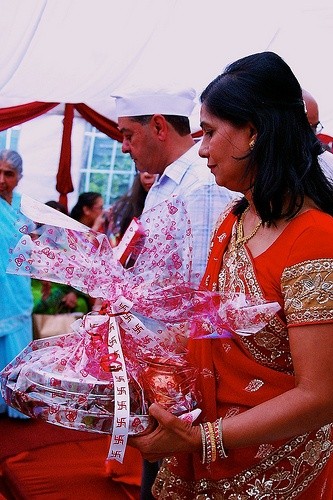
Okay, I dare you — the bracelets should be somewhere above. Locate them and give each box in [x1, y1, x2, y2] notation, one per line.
[199, 417, 228, 465]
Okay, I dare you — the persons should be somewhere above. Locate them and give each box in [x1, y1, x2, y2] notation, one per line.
[297, 88, 333, 186]
[0, 149, 37, 419]
[126, 51, 333, 500]
[31, 172, 159, 314]
[93, 83, 244, 322]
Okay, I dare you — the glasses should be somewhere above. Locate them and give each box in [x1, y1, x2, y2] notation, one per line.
[310, 121, 323, 132]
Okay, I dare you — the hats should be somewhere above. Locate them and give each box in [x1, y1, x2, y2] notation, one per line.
[112, 84, 197, 118]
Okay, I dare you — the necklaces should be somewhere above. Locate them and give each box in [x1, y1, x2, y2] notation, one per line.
[234, 205, 262, 249]
